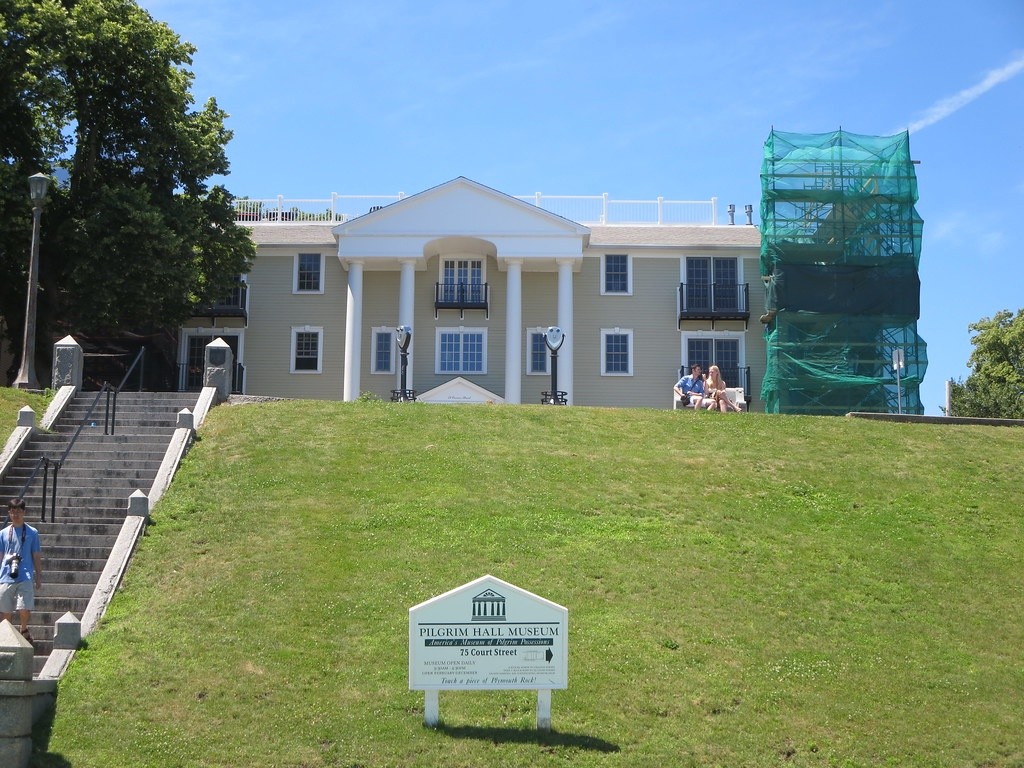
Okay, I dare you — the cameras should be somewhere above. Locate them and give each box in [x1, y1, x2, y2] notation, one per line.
[8, 553, 23, 578]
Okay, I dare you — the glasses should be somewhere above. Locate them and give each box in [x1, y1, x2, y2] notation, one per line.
[8, 510, 23, 516]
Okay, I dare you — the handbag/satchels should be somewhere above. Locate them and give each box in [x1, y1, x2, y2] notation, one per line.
[681, 394, 690, 406]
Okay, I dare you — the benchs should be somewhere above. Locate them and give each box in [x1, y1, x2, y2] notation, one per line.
[673, 386, 747, 413]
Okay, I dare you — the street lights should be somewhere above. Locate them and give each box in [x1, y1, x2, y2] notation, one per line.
[12, 171, 52, 392]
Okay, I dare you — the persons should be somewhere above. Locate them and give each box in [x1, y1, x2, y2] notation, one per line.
[674, 363, 715, 411]
[702, 366, 742, 412]
[0, 498, 41, 642]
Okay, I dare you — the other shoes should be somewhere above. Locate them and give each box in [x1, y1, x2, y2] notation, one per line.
[734, 407, 742, 412]
[20, 629, 34, 644]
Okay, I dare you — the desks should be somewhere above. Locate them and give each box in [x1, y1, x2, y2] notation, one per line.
[269, 211, 292, 221]
[233, 211, 261, 221]
